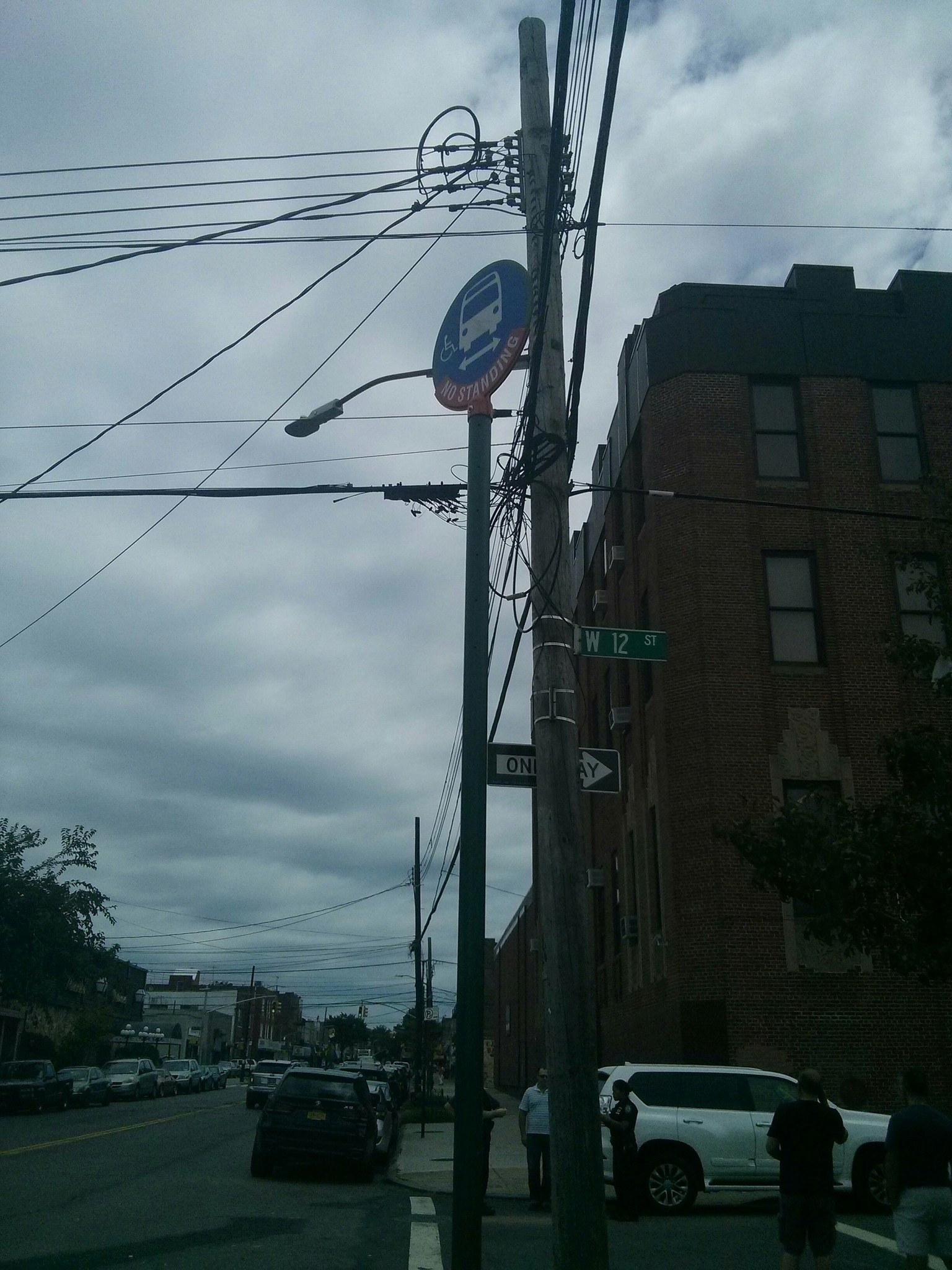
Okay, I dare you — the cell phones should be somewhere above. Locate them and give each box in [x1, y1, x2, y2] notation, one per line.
[815, 1080, 822, 1094]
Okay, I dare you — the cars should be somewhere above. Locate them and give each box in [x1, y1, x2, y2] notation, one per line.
[56, 1065, 112, 1107]
[154, 1068, 179, 1097]
[200, 1057, 256, 1093]
[325, 1055, 411, 1155]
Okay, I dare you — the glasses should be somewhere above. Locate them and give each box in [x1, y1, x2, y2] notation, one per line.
[538, 1075, 548, 1079]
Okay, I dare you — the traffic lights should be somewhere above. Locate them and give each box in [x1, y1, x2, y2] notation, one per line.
[362, 1006, 368, 1017]
[359, 1006, 363, 1017]
[270, 1000, 282, 1014]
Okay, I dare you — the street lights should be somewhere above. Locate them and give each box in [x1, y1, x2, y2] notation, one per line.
[137, 1025, 165, 1051]
[282, 351, 608, 1269]
[121, 1023, 136, 1051]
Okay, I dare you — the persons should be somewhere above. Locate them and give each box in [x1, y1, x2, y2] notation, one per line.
[765, 1069, 848, 1270]
[519, 1063, 553, 1211]
[885, 1067, 952, 1270]
[600, 1079, 640, 1222]
[443, 1077, 509, 1215]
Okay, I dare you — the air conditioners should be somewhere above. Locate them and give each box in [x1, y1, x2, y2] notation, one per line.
[608, 546, 625, 570]
[620, 916, 638, 940]
[609, 707, 630, 731]
[586, 869, 603, 888]
[529, 939, 538, 952]
[592, 590, 606, 613]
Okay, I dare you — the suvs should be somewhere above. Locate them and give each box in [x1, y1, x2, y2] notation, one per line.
[246, 1057, 303, 1109]
[593, 1060, 900, 1217]
[100, 1056, 158, 1101]
[250, 1063, 381, 1178]
[161, 1057, 203, 1095]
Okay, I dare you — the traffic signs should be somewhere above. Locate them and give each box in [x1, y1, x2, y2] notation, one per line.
[484, 740, 625, 796]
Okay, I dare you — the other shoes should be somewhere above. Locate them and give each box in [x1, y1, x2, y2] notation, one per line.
[530, 1199, 538, 1208]
[482, 1207, 496, 1214]
[540, 1201, 550, 1208]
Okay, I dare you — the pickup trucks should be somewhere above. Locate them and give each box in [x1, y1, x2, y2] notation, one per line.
[0, 1060, 75, 1113]
